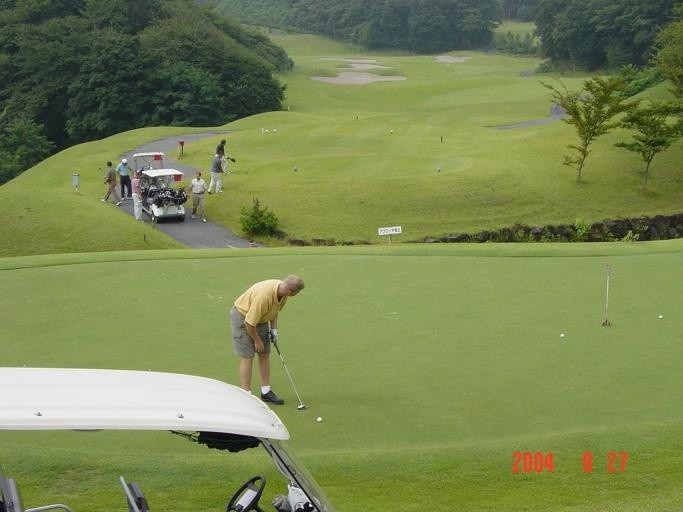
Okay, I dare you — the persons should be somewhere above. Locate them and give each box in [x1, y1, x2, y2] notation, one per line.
[116, 159, 135, 200]
[230, 274, 305, 405]
[208, 151, 225, 194]
[131, 169, 146, 221]
[187, 172, 208, 224]
[100, 161, 122, 205]
[216, 138, 229, 174]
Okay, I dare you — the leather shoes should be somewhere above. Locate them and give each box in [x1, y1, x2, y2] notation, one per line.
[260, 390, 283, 404]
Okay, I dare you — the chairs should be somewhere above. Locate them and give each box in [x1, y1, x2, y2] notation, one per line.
[120, 476, 151, 512]
[0, 476, 70, 511]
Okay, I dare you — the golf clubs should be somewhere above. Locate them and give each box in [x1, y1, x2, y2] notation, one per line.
[603, 264, 610, 327]
[269, 331, 306, 409]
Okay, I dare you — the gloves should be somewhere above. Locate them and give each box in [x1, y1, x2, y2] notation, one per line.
[268, 328, 277, 343]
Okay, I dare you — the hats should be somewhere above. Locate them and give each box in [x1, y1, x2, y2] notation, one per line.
[121, 159, 128, 164]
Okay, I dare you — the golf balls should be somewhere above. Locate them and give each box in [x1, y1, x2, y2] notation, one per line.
[560, 334, 564, 338]
[659, 316, 662, 317]
[317, 417, 321, 421]
